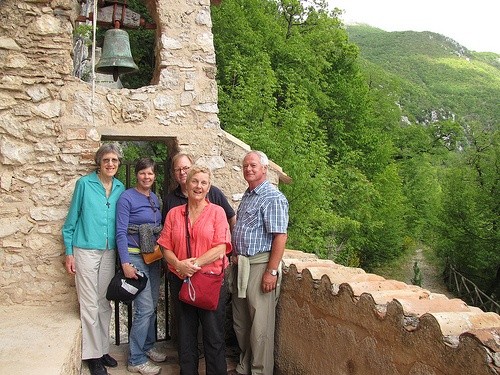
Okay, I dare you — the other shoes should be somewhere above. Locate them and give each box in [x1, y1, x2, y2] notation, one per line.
[100, 355, 117, 367]
[197, 349, 205, 359]
[88, 357, 107, 375]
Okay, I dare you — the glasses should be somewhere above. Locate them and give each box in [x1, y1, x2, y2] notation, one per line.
[148, 196, 157, 211]
[174, 166, 192, 173]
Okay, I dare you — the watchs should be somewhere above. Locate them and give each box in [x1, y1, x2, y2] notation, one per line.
[265, 268, 278, 276]
[193, 260, 199, 268]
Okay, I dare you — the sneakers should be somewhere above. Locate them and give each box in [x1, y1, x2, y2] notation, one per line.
[145, 347, 167, 362]
[127, 357, 162, 375]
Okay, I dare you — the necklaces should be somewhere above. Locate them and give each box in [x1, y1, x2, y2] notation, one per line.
[105, 200, 111, 208]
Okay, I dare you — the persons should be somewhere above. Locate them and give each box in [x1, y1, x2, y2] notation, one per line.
[156, 164, 233, 375]
[116, 157, 168, 375]
[61, 143, 126, 375]
[231, 151, 289, 375]
[160, 150, 235, 359]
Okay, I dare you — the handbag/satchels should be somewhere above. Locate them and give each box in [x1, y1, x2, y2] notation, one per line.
[142, 244, 163, 264]
[178, 270, 222, 311]
